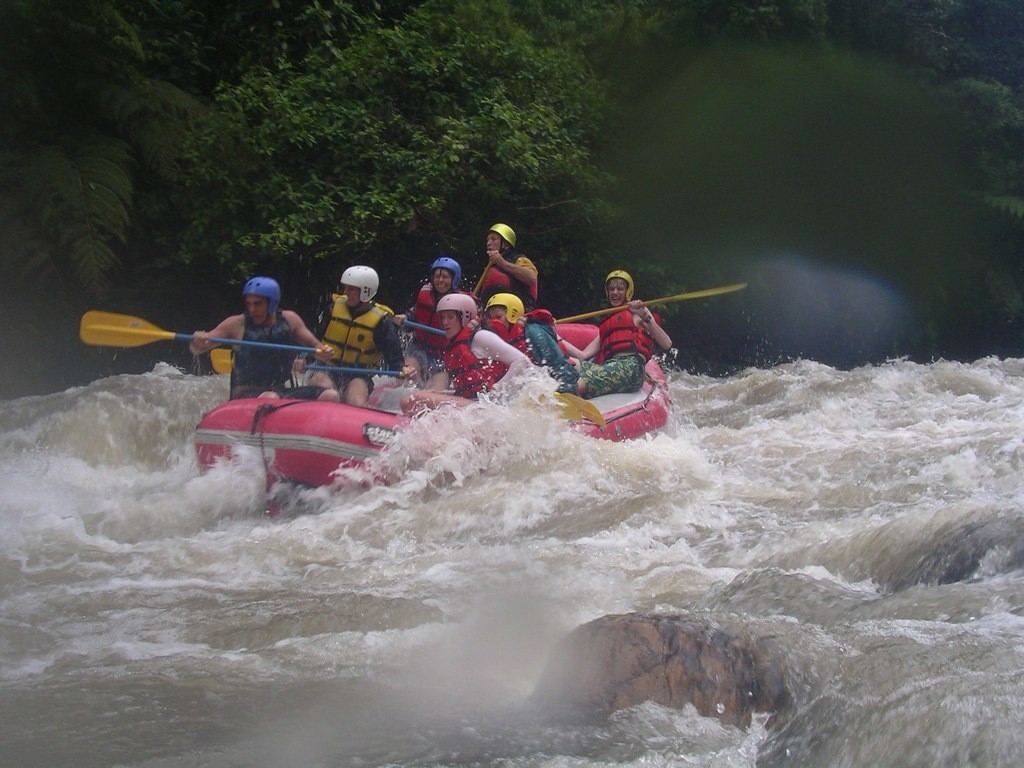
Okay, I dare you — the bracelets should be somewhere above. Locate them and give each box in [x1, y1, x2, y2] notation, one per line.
[642, 313, 654, 324]
[557, 338, 564, 344]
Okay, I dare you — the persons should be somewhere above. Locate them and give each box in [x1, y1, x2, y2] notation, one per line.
[189, 277, 340, 404]
[401, 293, 532, 424]
[556, 270, 672, 400]
[470, 223, 580, 395]
[392, 256, 461, 393]
[291, 265, 417, 408]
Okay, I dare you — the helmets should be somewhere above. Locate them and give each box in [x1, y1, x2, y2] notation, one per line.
[243, 277, 281, 313]
[431, 257, 462, 290]
[436, 293, 477, 327]
[605, 270, 634, 301]
[341, 265, 379, 302]
[484, 293, 525, 324]
[489, 223, 516, 248]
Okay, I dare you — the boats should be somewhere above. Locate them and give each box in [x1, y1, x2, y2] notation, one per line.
[195, 320, 670, 491]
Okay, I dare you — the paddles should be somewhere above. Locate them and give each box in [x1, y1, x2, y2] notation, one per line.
[331, 293, 447, 337]
[555, 281, 748, 323]
[79, 310, 329, 353]
[473, 258, 493, 296]
[505, 391, 606, 426]
[210, 349, 403, 377]
